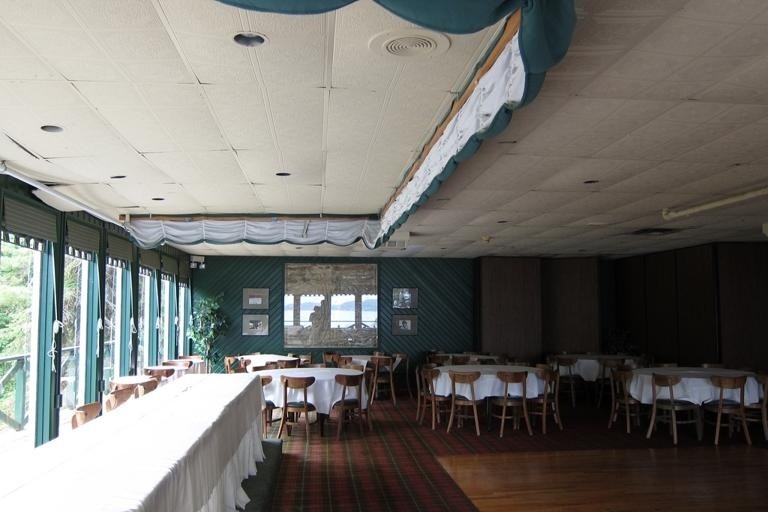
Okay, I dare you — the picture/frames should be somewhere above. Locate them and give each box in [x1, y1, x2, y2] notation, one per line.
[242, 315, 269, 335]
[242, 288, 269, 308]
[283, 261, 379, 349]
[391, 314, 417, 335]
[392, 287, 418, 308]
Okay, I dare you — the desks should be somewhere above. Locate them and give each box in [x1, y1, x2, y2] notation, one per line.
[115, 376, 151, 383]
[554, 356, 633, 408]
[0, 373, 261, 512]
[191, 360, 206, 373]
[632, 369, 755, 440]
[341, 355, 387, 399]
[256, 368, 362, 437]
[153, 366, 188, 375]
[236, 355, 297, 372]
[435, 366, 544, 434]
[437, 355, 498, 363]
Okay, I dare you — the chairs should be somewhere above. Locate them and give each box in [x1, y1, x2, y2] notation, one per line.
[428, 356, 443, 365]
[253, 366, 276, 371]
[646, 373, 703, 444]
[266, 362, 280, 368]
[505, 358, 528, 366]
[366, 357, 397, 406]
[322, 352, 338, 367]
[352, 365, 363, 371]
[446, 370, 485, 436]
[596, 358, 627, 411]
[416, 363, 435, 422]
[420, 368, 449, 430]
[110, 383, 134, 396]
[163, 361, 191, 369]
[527, 369, 563, 434]
[152, 369, 174, 379]
[374, 353, 385, 356]
[76, 402, 101, 426]
[334, 357, 353, 368]
[299, 355, 312, 367]
[261, 375, 275, 439]
[735, 372, 768, 440]
[179, 356, 204, 361]
[703, 376, 752, 448]
[480, 359, 495, 364]
[110, 388, 132, 408]
[349, 361, 377, 431]
[532, 364, 558, 425]
[234, 368, 247, 373]
[613, 365, 632, 422]
[308, 363, 326, 368]
[641, 354, 655, 367]
[278, 359, 300, 368]
[333, 374, 364, 441]
[393, 353, 413, 400]
[488, 371, 533, 437]
[320, 364, 335, 368]
[608, 368, 641, 433]
[557, 358, 577, 409]
[225, 357, 237, 373]
[276, 375, 316, 440]
[134, 379, 158, 399]
[239, 359, 251, 368]
[452, 355, 468, 365]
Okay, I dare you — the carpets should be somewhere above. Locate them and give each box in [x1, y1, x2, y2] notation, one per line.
[268, 388, 768, 512]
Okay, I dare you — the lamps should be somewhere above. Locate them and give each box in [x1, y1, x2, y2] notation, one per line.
[663, 186, 768, 222]
